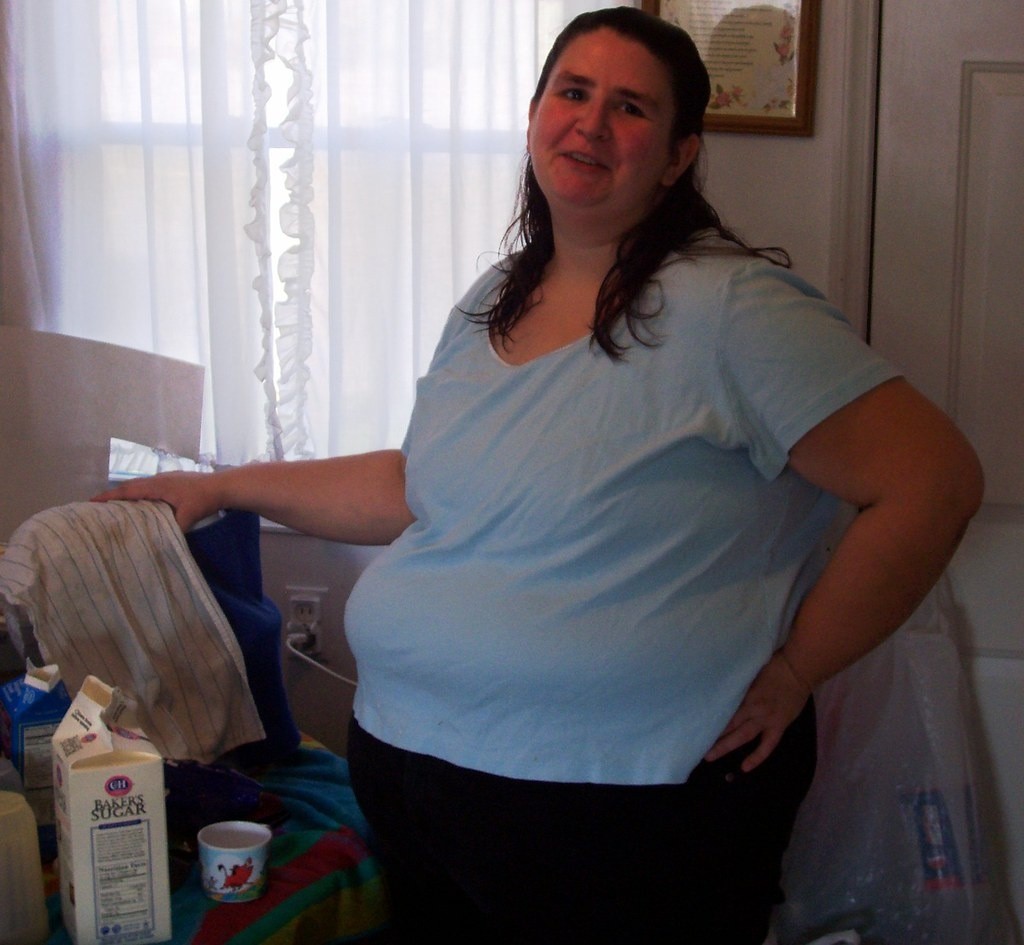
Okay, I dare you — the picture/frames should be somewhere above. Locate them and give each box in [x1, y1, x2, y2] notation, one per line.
[638, 1, 820, 138]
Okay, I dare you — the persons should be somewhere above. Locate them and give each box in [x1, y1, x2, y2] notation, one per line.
[88, 7, 984, 945]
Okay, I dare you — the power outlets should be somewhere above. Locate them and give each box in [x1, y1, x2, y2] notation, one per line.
[281, 587, 329, 663]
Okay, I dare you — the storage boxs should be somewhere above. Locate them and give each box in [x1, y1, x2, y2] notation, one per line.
[52, 671, 174, 945]
[0, 657, 73, 864]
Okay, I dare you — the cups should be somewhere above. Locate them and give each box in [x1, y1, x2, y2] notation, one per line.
[197, 819, 272, 904]
